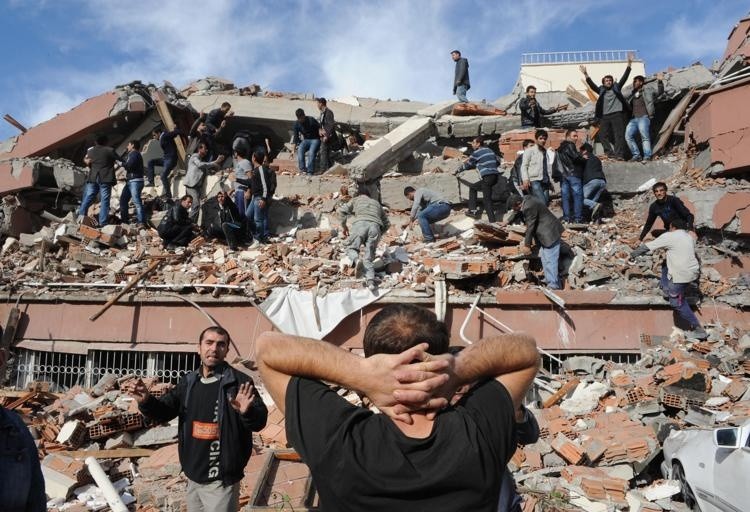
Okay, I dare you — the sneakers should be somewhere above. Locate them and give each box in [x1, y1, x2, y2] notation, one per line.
[354, 259, 364, 280]
[686, 327, 707, 340]
[591, 202, 603, 220]
[367, 280, 378, 290]
[464, 209, 479, 219]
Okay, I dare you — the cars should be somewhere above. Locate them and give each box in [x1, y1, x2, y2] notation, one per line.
[664, 419, 750, 511]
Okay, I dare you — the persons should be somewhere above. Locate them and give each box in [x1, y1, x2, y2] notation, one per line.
[0, 404, 48, 511]
[579, 51, 664, 163]
[334, 185, 385, 291]
[78, 98, 335, 251]
[500, 195, 565, 290]
[256, 302, 541, 512]
[632, 181, 709, 341]
[452, 50, 471, 103]
[401, 185, 452, 242]
[519, 86, 569, 128]
[444, 346, 539, 512]
[452, 135, 501, 223]
[128, 326, 267, 512]
[508, 130, 607, 224]
